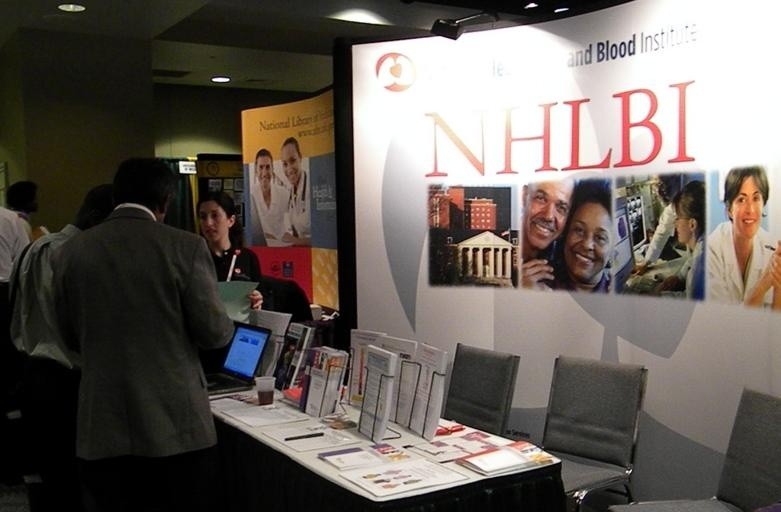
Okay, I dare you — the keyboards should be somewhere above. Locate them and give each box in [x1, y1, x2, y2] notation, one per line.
[634, 278, 660, 296]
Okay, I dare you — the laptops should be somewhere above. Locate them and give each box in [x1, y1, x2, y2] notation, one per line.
[204, 323, 272, 396]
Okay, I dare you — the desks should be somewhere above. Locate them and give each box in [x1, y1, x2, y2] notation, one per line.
[209, 388, 566, 512]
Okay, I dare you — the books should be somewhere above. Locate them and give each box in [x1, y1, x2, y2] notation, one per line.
[318, 447, 384, 471]
[455, 440, 554, 477]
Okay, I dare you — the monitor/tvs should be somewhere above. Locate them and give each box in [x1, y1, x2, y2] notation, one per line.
[614, 195, 636, 294]
[627, 195, 647, 255]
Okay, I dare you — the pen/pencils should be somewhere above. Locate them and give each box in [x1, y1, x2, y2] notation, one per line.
[285, 433, 324, 441]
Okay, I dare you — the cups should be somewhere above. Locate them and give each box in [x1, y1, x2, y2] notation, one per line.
[255, 376, 276, 405]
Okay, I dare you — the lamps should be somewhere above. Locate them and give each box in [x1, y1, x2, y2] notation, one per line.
[57, 2, 88, 18]
[428, 15, 501, 42]
[209, 73, 232, 88]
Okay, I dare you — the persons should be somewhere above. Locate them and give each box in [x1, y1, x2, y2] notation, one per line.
[196, 190, 264, 313]
[281, 137, 312, 247]
[631, 175, 682, 277]
[249, 147, 294, 249]
[550, 178, 613, 295]
[8, 182, 118, 512]
[0, 206, 31, 412]
[7, 179, 38, 244]
[655, 179, 706, 300]
[54, 155, 235, 460]
[520, 179, 576, 290]
[705, 166, 781, 310]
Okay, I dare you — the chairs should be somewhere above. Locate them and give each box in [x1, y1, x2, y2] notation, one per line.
[608, 385, 779, 511]
[440, 344, 520, 434]
[538, 355, 650, 510]
